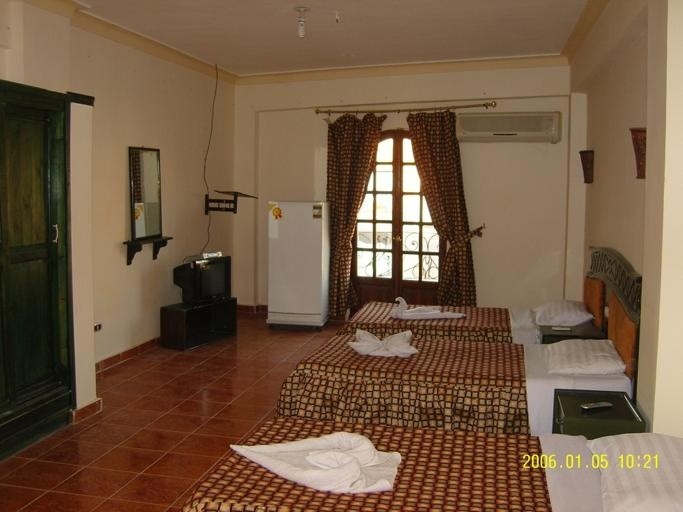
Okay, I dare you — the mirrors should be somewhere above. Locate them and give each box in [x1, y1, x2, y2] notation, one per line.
[128, 145, 162, 240]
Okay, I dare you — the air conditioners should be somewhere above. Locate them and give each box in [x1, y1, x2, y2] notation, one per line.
[455, 112, 562, 143]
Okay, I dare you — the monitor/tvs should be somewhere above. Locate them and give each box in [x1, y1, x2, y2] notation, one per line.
[173, 256, 231, 303]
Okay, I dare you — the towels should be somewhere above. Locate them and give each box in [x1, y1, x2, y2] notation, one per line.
[228, 430, 401, 493]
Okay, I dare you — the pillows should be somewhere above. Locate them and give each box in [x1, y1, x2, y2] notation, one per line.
[586, 433, 683, 512]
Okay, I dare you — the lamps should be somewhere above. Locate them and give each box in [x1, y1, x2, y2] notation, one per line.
[295, 6, 309, 39]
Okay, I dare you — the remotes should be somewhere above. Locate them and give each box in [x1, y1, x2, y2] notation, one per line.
[581, 401, 614, 411]
[552, 327, 571, 331]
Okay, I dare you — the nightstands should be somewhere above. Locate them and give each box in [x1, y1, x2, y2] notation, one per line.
[551, 388, 646, 439]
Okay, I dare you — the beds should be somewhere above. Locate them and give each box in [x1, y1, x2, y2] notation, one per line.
[179, 415, 683, 512]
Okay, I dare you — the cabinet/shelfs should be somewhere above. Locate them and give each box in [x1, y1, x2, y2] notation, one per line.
[160, 297, 238, 352]
